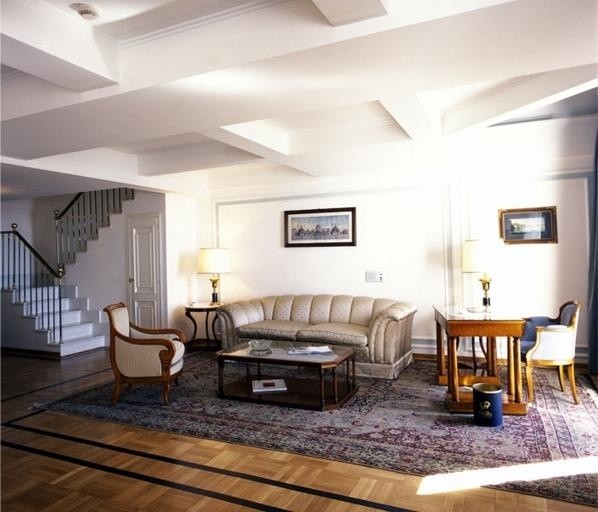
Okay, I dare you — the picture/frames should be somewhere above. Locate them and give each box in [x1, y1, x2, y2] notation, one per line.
[283, 207, 356, 247]
[499, 206, 558, 244]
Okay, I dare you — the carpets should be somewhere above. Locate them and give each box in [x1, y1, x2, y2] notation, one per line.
[32, 339, 598, 508]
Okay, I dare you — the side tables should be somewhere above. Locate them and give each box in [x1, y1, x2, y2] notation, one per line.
[185, 302, 225, 353]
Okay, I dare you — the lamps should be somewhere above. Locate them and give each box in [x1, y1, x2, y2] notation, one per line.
[462, 239, 505, 313]
[196, 248, 233, 306]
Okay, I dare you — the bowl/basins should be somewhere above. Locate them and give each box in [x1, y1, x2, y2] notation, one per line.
[248, 349, 273, 356]
[247, 339, 272, 350]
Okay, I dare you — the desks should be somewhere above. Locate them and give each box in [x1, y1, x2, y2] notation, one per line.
[432, 304, 528, 417]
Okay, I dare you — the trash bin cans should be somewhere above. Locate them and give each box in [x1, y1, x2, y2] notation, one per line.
[471, 382, 503, 427]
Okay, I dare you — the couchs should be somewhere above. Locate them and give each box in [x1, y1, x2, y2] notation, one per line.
[215, 295, 417, 380]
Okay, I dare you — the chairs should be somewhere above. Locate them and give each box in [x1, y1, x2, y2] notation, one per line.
[519, 300, 583, 406]
[102, 302, 187, 406]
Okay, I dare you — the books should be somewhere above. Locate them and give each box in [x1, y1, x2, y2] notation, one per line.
[252, 379, 288, 392]
